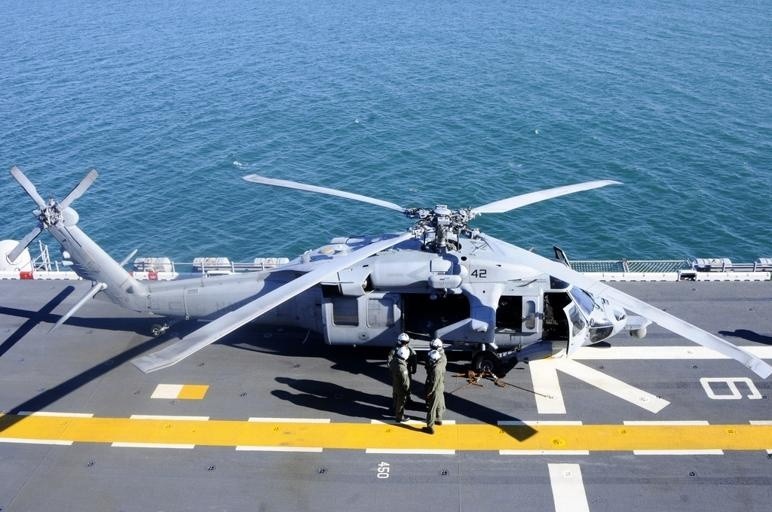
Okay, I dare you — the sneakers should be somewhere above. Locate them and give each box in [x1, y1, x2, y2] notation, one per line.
[396, 416, 410, 423]
[435, 421, 442, 425]
[421, 427, 433, 434]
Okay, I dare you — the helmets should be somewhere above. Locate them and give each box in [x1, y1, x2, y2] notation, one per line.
[427, 349, 440, 361]
[397, 332, 409, 345]
[430, 338, 442, 349]
[397, 345, 411, 360]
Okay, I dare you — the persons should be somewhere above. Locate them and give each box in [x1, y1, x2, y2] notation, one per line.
[422, 350, 443, 434]
[389, 346, 411, 422]
[426, 338, 447, 411]
[387, 333, 417, 406]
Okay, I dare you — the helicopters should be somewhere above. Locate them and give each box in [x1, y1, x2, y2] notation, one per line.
[3, 161, 772, 385]
